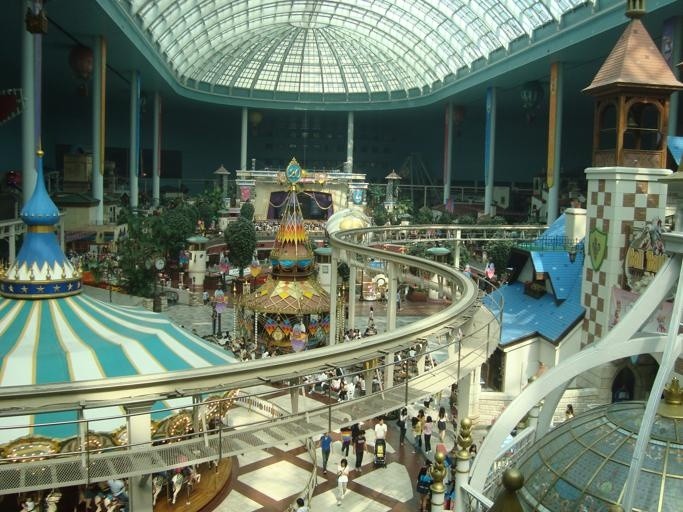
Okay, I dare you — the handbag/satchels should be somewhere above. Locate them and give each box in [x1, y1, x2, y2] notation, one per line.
[396, 418, 405, 428]
[416, 480, 430, 494]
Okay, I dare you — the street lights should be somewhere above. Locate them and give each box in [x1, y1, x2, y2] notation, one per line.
[103, 255, 119, 302]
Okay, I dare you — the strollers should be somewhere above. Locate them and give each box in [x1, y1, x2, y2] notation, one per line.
[372, 436, 390, 467]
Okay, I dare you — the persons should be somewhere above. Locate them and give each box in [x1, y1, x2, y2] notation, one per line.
[385, 289, 403, 312]
[565, 402, 573, 420]
[342, 306, 374, 342]
[0, 438, 216, 512]
[215, 330, 281, 358]
[299, 344, 455, 510]
[252, 214, 326, 232]
